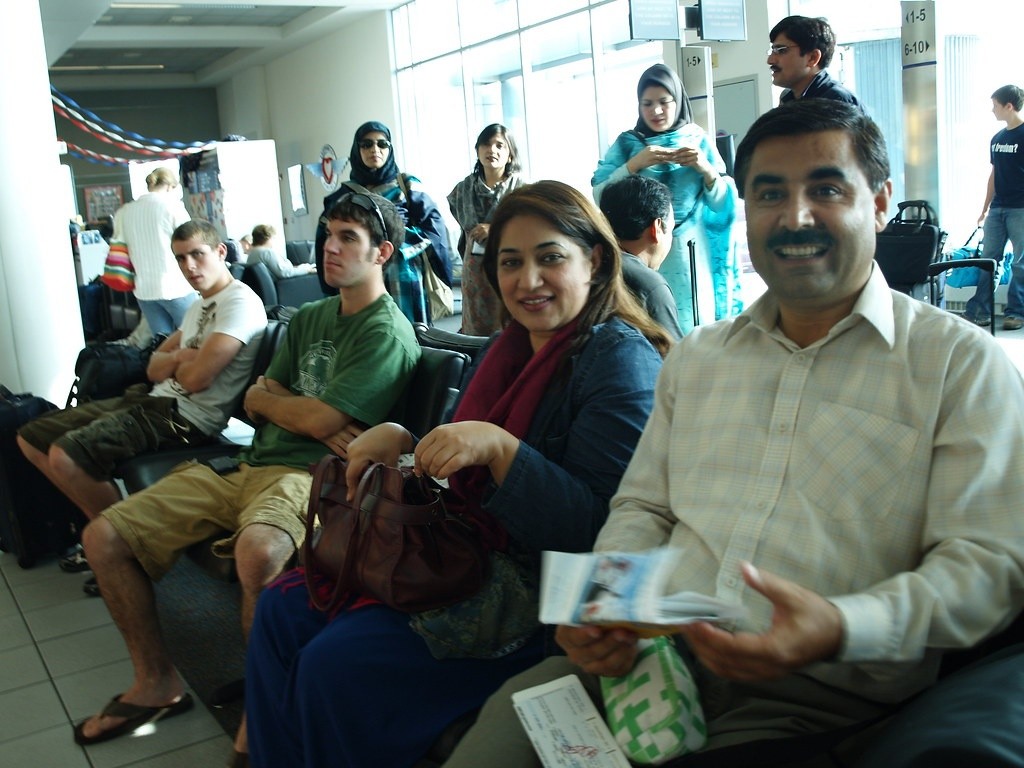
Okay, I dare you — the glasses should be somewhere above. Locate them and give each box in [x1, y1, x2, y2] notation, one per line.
[767, 44, 800, 56]
[330, 191, 388, 242]
[358, 137, 391, 150]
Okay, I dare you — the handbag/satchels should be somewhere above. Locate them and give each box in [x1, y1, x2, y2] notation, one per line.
[299, 453, 487, 613]
[877, 201, 939, 291]
[945, 226, 1013, 287]
[101, 201, 136, 292]
[424, 263, 454, 322]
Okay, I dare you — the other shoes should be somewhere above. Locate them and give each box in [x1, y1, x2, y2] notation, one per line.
[82, 577, 101, 597]
[59, 548, 91, 573]
[960, 312, 992, 326]
[1003, 316, 1024, 330]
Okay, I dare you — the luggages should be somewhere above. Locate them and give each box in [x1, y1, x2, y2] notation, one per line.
[0, 385, 91, 569]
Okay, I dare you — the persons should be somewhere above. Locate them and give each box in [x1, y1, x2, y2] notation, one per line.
[16, 219, 269, 595]
[68, 189, 422, 768]
[959, 84, 1024, 331]
[766, 14, 873, 119]
[238, 233, 254, 254]
[432, 94, 1024, 768]
[117, 165, 203, 338]
[312, 119, 453, 329]
[596, 171, 686, 342]
[245, 224, 317, 281]
[68, 215, 84, 234]
[590, 62, 750, 339]
[238, 177, 675, 768]
[222, 237, 243, 279]
[445, 123, 529, 337]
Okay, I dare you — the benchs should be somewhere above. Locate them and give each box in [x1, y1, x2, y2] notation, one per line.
[141, 304, 488, 745]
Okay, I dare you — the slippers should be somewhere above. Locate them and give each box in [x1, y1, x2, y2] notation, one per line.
[227, 747, 250, 768]
[74, 692, 195, 745]
